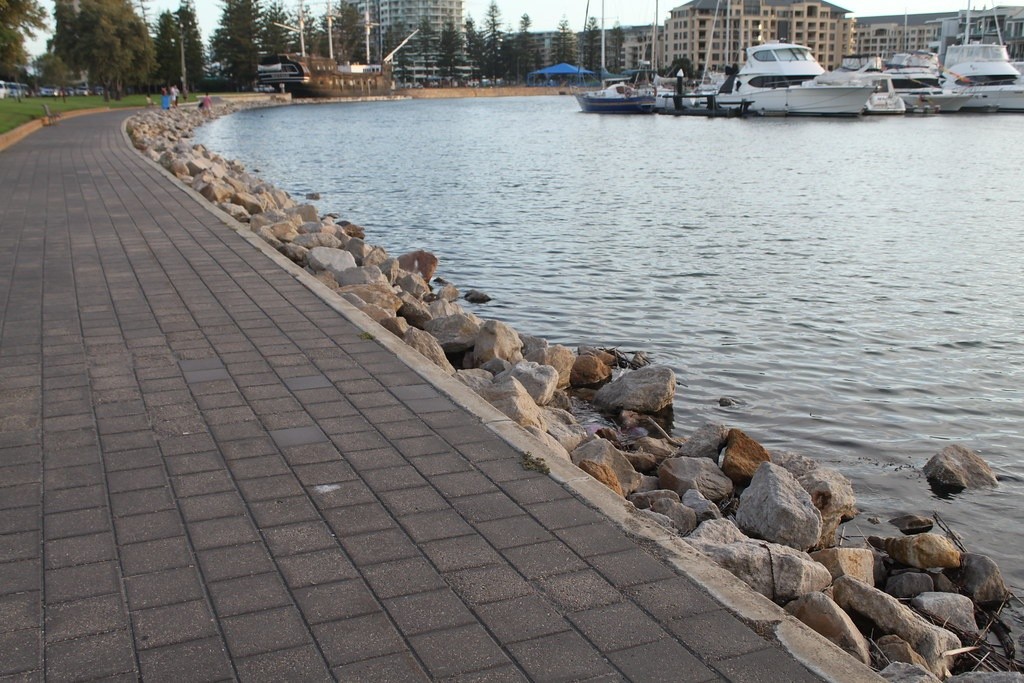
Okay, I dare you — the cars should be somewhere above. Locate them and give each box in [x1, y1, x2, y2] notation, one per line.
[0, 81, 105, 98]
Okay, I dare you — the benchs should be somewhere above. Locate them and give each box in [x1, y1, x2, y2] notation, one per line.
[41, 103, 62, 125]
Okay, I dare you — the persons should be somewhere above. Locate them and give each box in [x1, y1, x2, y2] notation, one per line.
[203, 92, 213, 119]
[161, 84, 204, 110]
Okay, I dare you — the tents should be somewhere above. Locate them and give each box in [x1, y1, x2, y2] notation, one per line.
[526, 62, 597, 87]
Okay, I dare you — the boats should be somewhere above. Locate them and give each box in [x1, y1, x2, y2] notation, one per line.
[256, 0, 419, 97]
[640, 0, 1024, 115]
[575, 0, 656, 114]
